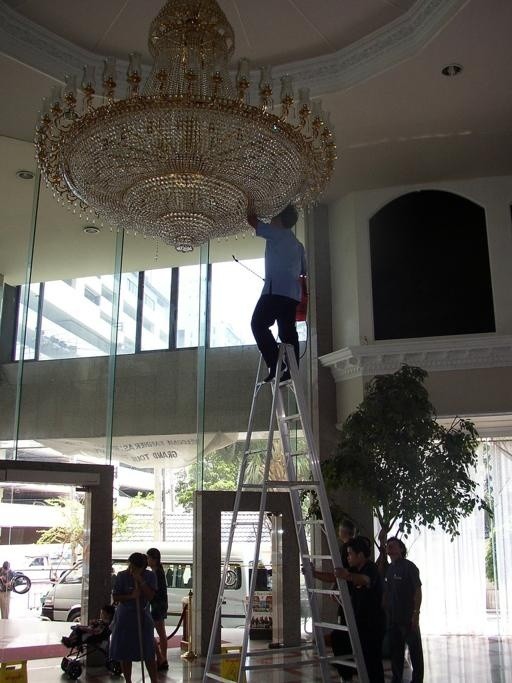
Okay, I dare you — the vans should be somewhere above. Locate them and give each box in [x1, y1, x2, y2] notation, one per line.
[41, 556, 273, 628]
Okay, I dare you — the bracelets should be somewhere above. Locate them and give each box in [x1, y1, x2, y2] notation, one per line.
[412, 609, 421, 613]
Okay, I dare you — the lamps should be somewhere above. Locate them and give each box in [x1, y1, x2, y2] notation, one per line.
[31, 0, 341, 256]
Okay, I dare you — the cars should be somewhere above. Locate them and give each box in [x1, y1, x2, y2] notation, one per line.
[14, 554, 82, 583]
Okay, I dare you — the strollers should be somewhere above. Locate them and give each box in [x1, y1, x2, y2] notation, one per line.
[62, 600, 121, 681]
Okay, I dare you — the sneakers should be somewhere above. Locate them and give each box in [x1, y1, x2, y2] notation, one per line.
[263, 363, 291, 385]
[158, 660, 169, 672]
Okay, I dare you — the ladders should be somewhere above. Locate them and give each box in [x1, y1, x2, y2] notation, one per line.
[203, 343, 369, 683]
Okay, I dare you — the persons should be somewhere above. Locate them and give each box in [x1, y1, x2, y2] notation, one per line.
[109, 552, 159, 683]
[49, 578, 58, 586]
[60, 603, 114, 649]
[301, 539, 386, 683]
[0, 562, 16, 620]
[333, 519, 356, 622]
[245, 198, 309, 385]
[384, 537, 424, 683]
[145, 549, 169, 669]
